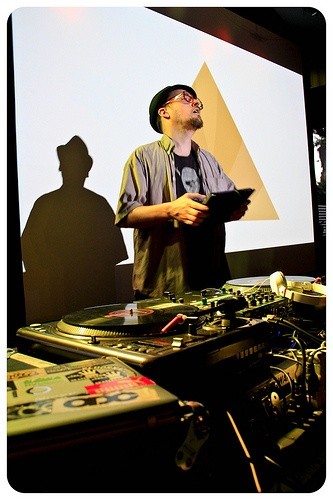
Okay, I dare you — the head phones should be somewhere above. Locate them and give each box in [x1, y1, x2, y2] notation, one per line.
[271, 271, 326, 307]
[173, 398, 224, 488]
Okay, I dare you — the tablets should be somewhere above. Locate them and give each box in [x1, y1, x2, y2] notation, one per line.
[198, 189, 254, 223]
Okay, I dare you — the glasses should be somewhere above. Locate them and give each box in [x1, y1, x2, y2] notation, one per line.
[161, 91, 204, 110]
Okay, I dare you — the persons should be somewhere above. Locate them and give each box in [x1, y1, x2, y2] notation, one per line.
[114, 83, 251, 301]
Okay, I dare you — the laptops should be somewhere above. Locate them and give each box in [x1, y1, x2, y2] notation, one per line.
[7, 356, 178, 443]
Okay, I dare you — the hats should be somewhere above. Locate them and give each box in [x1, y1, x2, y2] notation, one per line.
[149, 84, 197, 134]
